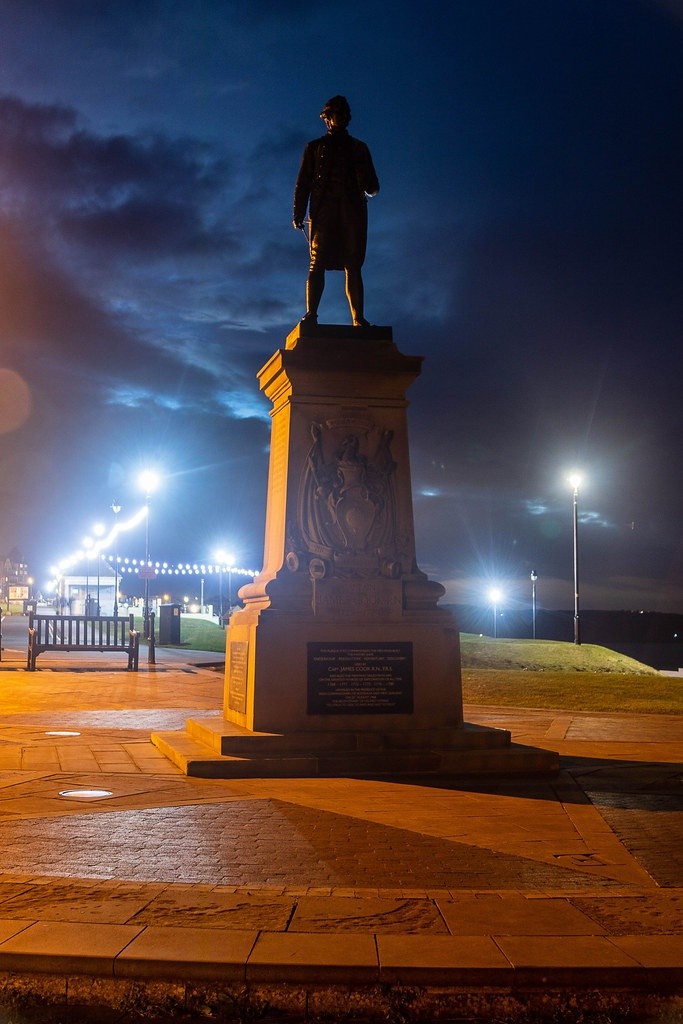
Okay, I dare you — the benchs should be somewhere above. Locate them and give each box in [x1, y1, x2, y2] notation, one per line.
[27, 611, 141, 673]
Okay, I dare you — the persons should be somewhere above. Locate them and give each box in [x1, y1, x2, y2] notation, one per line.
[293, 95, 380, 327]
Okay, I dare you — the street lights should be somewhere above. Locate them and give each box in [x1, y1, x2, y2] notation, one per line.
[110, 500, 123, 617]
[568, 472, 584, 646]
[94, 524, 105, 616]
[218, 551, 234, 616]
[492, 593, 498, 637]
[84, 537, 92, 615]
[139, 476, 157, 638]
[531, 570, 538, 639]
